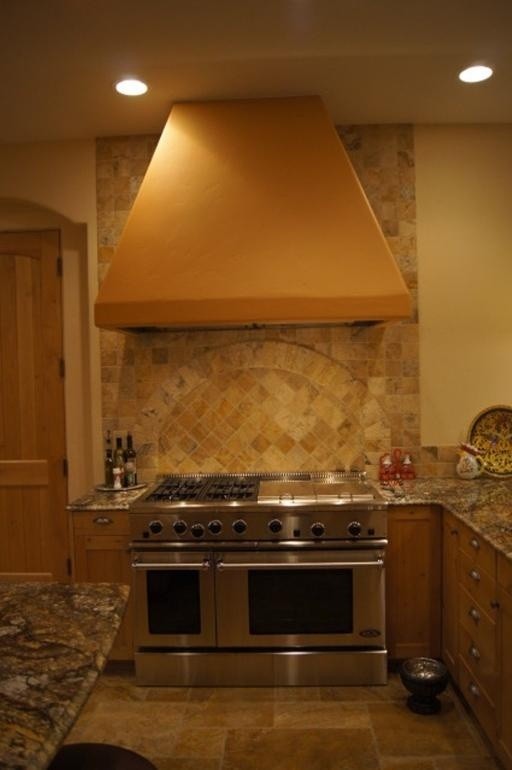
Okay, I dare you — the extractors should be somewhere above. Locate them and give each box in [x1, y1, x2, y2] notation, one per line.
[92, 93, 416, 340]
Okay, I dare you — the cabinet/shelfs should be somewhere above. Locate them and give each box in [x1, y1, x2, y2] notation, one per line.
[390, 506, 512, 769]
[71, 511, 131, 660]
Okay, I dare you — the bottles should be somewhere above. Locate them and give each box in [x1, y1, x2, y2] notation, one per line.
[105, 431, 137, 489]
[381, 452, 414, 481]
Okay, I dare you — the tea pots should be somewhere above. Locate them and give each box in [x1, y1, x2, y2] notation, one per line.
[456, 439, 483, 481]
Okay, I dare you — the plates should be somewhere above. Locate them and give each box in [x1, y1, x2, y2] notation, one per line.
[466, 404, 512, 478]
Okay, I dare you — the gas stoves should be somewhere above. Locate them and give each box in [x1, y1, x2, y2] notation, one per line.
[144, 473, 262, 506]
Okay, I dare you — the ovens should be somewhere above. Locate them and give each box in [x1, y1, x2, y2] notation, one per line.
[132, 548, 386, 651]
